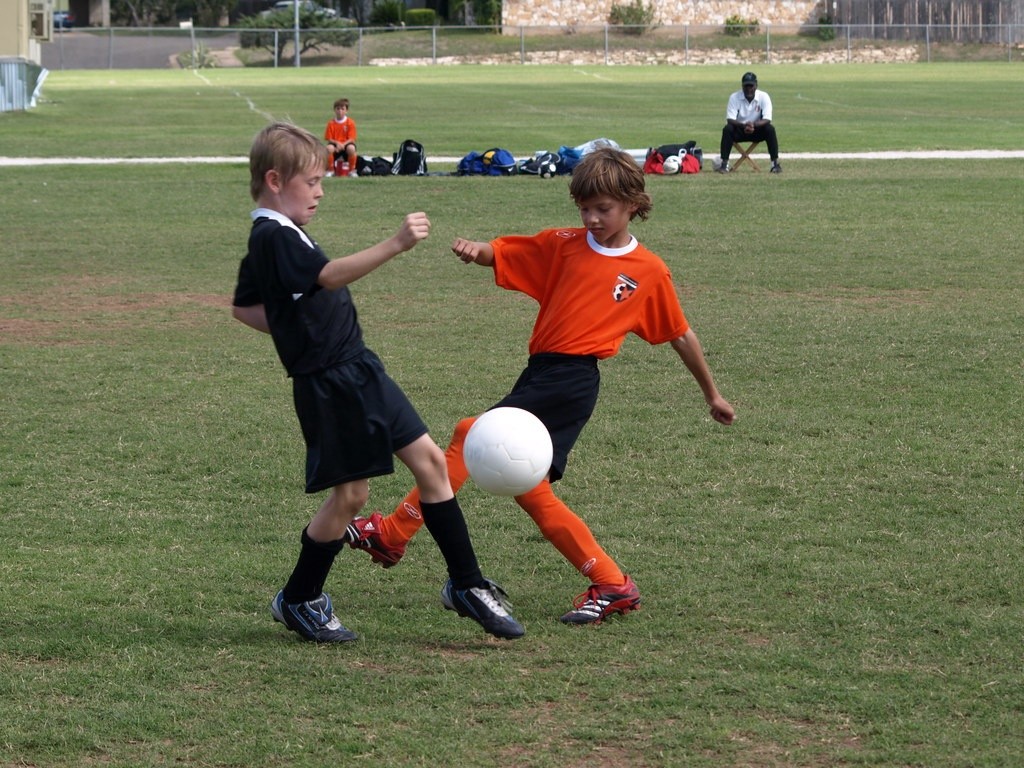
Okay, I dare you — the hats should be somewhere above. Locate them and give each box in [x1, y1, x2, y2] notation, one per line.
[742, 73, 757, 86]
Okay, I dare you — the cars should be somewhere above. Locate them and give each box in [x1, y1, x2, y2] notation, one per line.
[258, 0, 336, 25]
[52, 11, 73, 28]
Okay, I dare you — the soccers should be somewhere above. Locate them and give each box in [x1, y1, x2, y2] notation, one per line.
[538, 161, 557, 179]
[663, 155, 682, 175]
[463, 407, 555, 497]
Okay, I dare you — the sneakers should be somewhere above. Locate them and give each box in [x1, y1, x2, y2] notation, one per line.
[560, 572, 642, 626]
[270, 587, 357, 644]
[345, 512, 406, 570]
[440, 575, 526, 639]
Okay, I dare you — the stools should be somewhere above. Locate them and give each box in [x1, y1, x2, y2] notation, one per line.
[729, 141, 764, 173]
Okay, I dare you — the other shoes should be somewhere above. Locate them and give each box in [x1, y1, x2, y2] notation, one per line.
[719, 162, 729, 173]
[771, 163, 782, 174]
[348, 171, 359, 177]
[326, 171, 335, 177]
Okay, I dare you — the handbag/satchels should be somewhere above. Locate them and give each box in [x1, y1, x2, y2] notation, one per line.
[643, 150, 700, 175]
[458, 148, 516, 176]
[371, 157, 390, 176]
[518, 153, 559, 175]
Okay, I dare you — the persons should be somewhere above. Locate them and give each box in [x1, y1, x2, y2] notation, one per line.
[323, 99, 360, 178]
[345, 149, 737, 624]
[230, 122, 527, 641]
[719, 72, 782, 173]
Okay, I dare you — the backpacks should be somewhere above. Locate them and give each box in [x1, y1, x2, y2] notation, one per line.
[391, 139, 428, 175]
